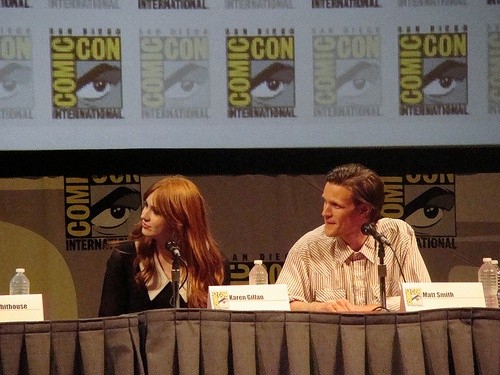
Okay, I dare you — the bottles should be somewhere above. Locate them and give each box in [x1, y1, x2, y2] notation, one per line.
[249, 260, 268, 285]
[490, 260, 500, 308]
[9, 268, 30, 294]
[477, 258, 499, 308]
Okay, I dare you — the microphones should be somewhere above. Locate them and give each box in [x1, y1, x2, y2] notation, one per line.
[361, 222, 391, 246]
[165, 239, 181, 258]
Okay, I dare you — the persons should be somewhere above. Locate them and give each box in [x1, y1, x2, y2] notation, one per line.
[274, 165, 432, 314]
[97, 176, 224, 317]
